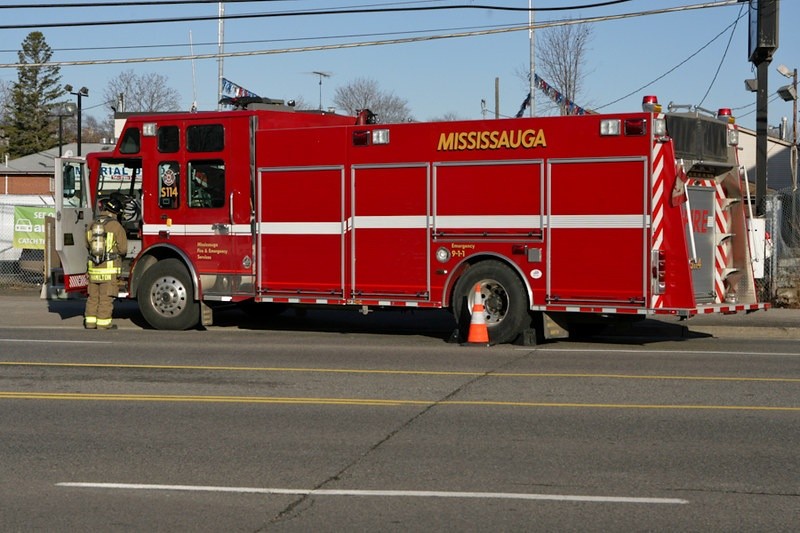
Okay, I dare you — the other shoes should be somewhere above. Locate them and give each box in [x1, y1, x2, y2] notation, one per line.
[97, 325, 118, 330]
[86, 323, 97, 328]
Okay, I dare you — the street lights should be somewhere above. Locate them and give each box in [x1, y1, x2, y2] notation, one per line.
[313, 71, 330, 112]
[777, 64, 798, 248]
[63, 84, 90, 156]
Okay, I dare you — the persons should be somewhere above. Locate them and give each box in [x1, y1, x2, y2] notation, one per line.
[85, 199, 127, 330]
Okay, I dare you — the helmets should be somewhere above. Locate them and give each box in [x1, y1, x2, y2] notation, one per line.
[104, 198, 121, 214]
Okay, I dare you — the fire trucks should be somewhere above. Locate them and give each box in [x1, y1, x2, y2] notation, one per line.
[38, 92, 772, 345]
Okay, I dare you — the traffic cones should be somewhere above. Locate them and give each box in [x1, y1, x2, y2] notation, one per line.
[466, 283, 490, 347]
